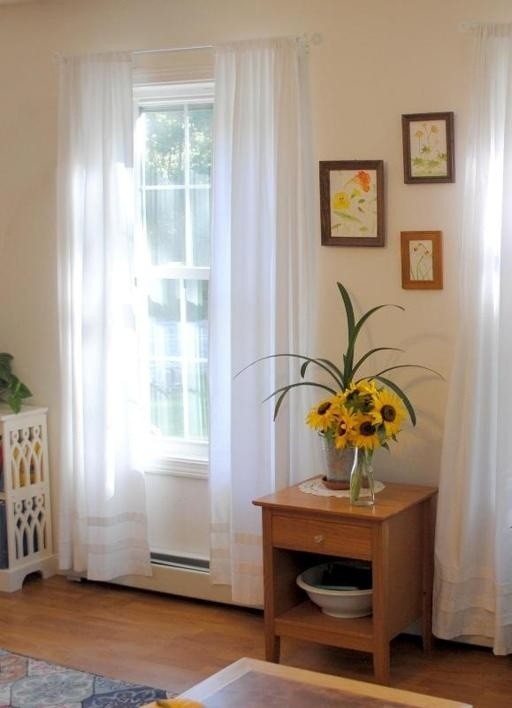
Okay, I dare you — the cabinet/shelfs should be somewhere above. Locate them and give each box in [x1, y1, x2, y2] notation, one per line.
[252, 475, 438, 686]
[0, 404, 55, 592]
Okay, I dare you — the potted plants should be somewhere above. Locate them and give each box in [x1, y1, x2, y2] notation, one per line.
[233, 282, 445, 491]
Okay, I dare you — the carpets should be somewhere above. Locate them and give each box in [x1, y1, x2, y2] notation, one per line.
[0, 648, 177, 708]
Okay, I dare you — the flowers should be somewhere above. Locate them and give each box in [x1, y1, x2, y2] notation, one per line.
[306, 378, 406, 502]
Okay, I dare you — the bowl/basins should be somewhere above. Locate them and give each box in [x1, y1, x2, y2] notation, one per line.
[295, 560, 373, 620]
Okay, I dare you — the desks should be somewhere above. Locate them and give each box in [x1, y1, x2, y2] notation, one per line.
[174, 657, 474, 707]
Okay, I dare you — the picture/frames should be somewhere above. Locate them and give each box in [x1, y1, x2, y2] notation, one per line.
[400, 231, 443, 289]
[401, 112, 456, 184]
[319, 160, 385, 247]
